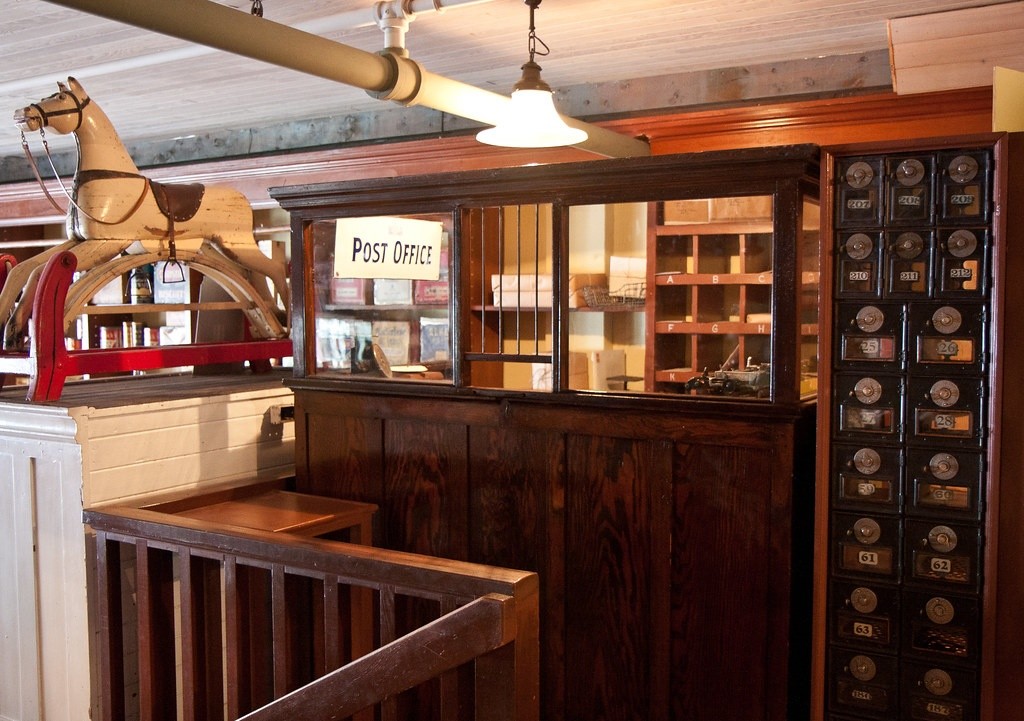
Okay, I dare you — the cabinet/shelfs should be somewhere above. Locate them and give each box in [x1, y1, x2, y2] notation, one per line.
[0, 364, 296, 721]
[172, 489, 376, 721]
[282, 376, 793, 721]
[809, 131, 1008, 721]
[268, 142, 822, 417]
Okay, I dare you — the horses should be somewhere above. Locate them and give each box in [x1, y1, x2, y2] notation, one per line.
[0, 76, 293, 350]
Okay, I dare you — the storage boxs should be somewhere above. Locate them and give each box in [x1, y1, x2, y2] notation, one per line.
[490, 273, 574, 309]
[532, 349, 589, 390]
[577, 281, 647, 306]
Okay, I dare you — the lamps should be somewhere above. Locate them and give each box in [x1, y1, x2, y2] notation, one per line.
[475, 0, 588, 149]
